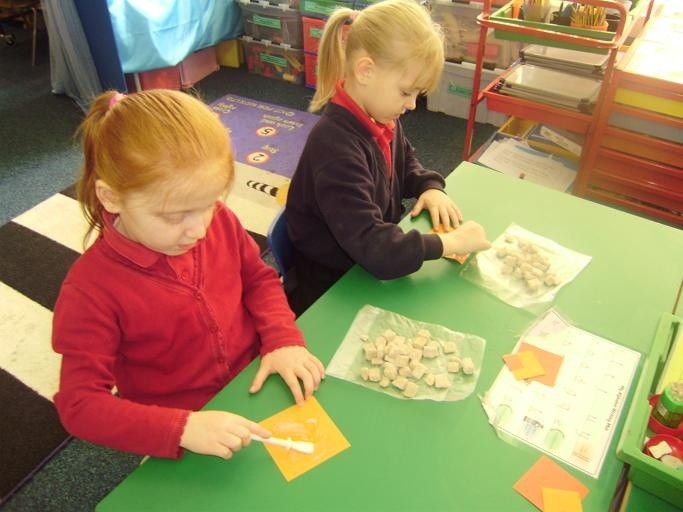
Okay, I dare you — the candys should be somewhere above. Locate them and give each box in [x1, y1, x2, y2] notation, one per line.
[360, 329, 473, 397]
[498, 238, 558, 290]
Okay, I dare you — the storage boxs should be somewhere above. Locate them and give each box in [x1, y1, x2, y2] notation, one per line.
[236, 0, 510, 130]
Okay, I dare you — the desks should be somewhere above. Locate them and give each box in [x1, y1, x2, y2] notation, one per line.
[95, 156, 683, 512]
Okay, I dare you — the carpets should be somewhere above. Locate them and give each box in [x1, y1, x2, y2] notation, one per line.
[1, 95, 322, 504]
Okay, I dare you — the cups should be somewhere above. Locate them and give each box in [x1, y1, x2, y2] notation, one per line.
[604, 13, 621, 32]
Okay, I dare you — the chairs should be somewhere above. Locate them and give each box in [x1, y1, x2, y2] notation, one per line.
[0, 0, 41, 66]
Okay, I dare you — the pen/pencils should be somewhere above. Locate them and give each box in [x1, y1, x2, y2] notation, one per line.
[571, 5, 607, 26]
[521, 1, 551, 13]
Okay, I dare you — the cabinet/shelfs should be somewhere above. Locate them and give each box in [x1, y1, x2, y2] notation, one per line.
[579, 6, 683, 228]
[463, 1, 653, 194]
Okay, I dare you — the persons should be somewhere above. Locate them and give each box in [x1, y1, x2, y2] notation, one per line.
[272, 2, 497, 315]
[49, 88, 327, 462]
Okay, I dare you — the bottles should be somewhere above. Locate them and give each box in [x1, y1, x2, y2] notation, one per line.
[653, 384, 683, 428]
